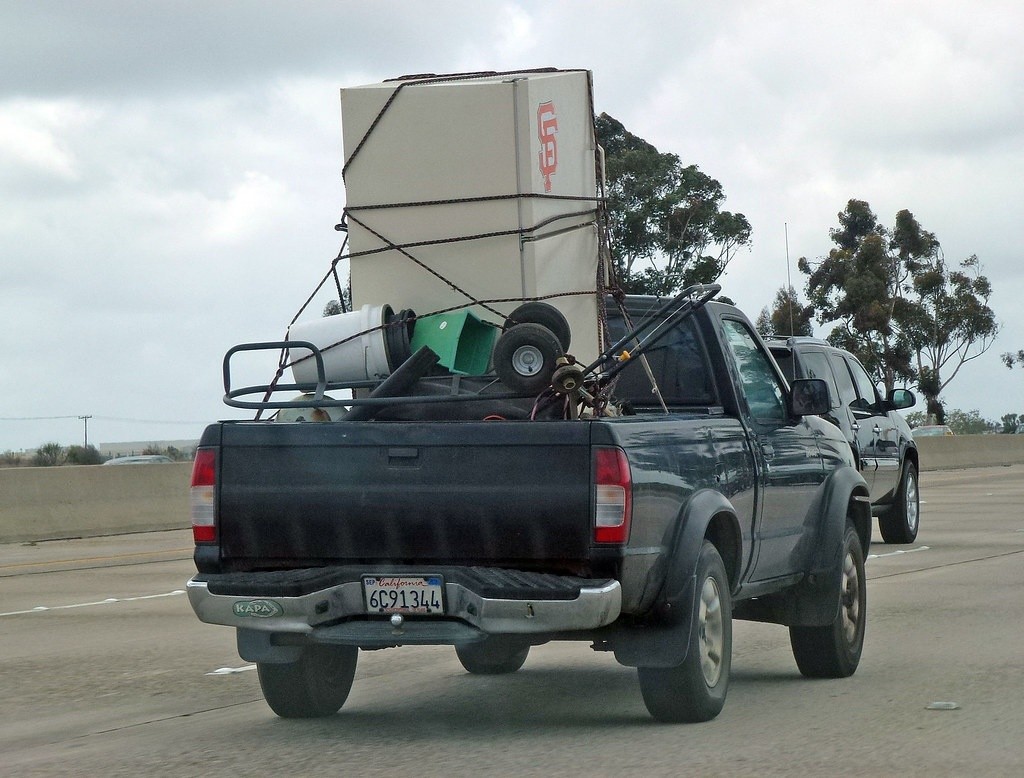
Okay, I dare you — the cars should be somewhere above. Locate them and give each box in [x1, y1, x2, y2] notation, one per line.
[909, 425, 953, 437]
[103, 454, 174, 464]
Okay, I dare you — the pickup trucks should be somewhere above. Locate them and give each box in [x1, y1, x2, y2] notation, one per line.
[184, 282, 875, 720]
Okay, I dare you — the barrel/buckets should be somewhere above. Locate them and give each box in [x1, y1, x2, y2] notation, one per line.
[288, 304, 417, 392]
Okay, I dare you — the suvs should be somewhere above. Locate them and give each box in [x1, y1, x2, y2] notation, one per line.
[761, 336, 921, 545]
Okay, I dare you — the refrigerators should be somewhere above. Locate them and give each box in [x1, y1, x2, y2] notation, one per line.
[340, 69, 609, 419]
